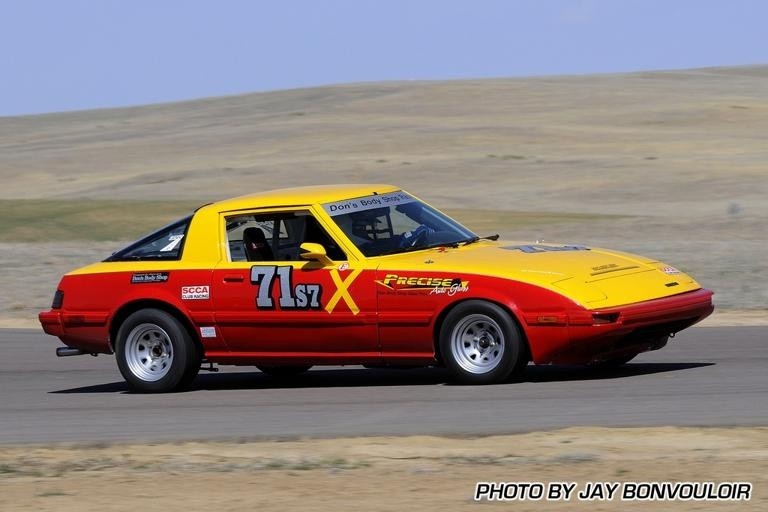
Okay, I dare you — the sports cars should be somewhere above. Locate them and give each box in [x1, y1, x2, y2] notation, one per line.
[37, 183, 716, 395]
